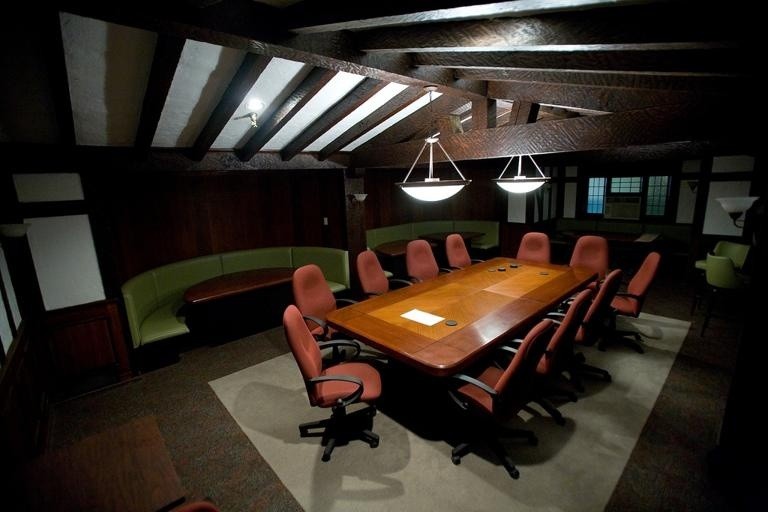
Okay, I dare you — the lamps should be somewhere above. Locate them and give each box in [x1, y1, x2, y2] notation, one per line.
[396, 85, 477, 203]
[488, 153, 554, 196]
[348, 192, 368, 211]
[713, 196, 760, 239]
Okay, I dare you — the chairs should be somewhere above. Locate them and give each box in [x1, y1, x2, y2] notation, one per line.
[684, 229, 751, 301]
[282, 220, 665, 480]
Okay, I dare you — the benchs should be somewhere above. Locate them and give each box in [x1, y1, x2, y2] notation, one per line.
[122, 244, 354, 349]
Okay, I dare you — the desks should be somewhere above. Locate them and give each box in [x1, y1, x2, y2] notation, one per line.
[181, 266, 301, 306]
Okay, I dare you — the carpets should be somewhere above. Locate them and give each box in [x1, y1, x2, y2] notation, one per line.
[204, 310, 695, 511]
